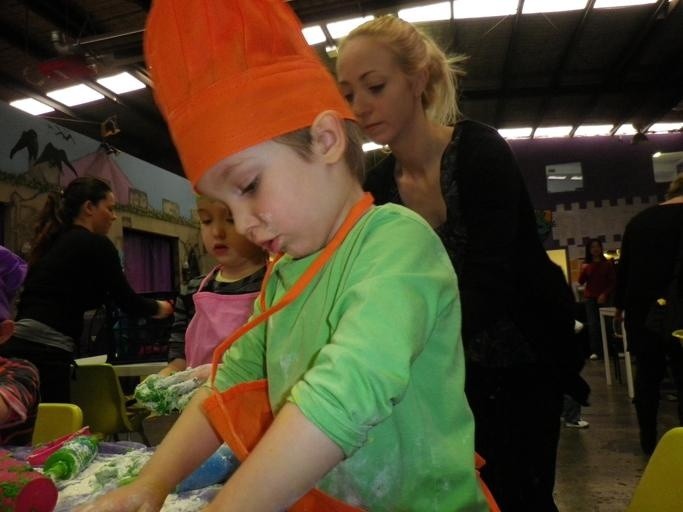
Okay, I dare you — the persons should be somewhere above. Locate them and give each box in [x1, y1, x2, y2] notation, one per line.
[0, 244, 40, 450]
[0, 175, 172, 416]
[618, 171, 683, 462]
[79, 108, 489, 510]
[331, 13, 580, 511]
[561, 378, 592, 435]
[576, 235, 621, 363]
[156, 188, 267, 380]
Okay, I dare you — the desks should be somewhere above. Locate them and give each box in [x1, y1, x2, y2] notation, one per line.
[599, 304, 634, 400]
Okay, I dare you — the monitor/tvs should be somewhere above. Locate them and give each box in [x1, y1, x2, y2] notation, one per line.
[546, 245, 571, 285]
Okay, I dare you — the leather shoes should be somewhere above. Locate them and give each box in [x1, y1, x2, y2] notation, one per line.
[560, 414, 590, 429]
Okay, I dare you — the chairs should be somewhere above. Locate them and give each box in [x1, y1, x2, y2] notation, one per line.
[30, 364, 146, 448]
[629, 424, 682, 510]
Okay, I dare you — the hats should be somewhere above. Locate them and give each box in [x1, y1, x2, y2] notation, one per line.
[0, 243, 30, 323]
[142, 0, 362, 196]
